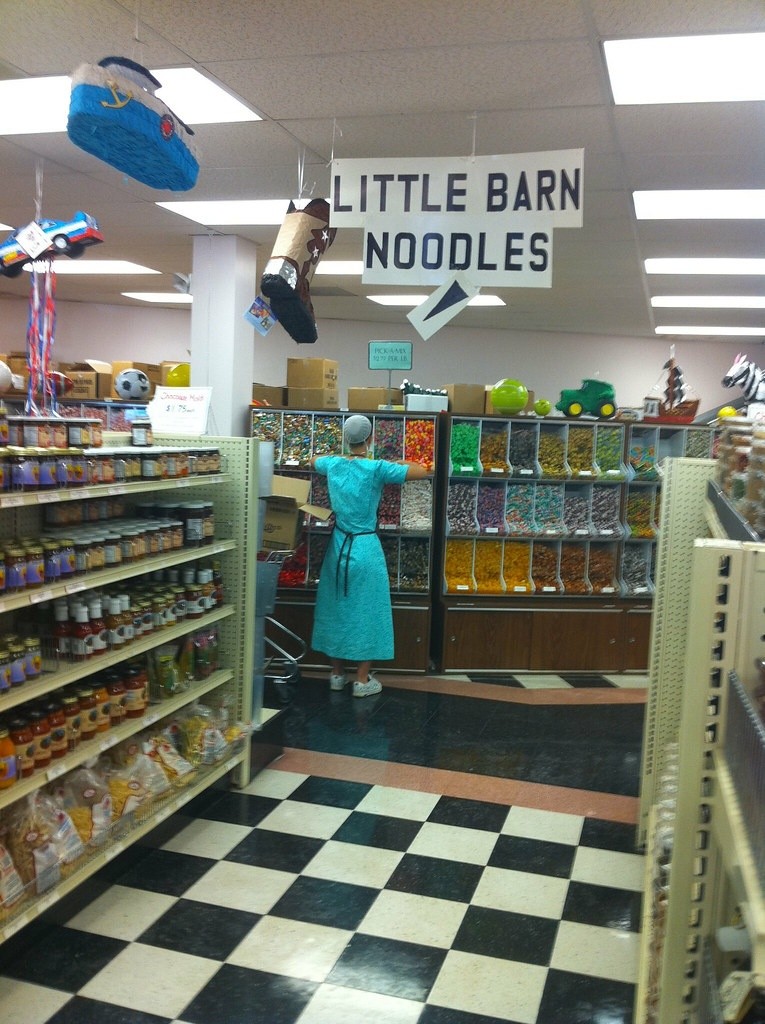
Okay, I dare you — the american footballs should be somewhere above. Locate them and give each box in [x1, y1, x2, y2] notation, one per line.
[34, 371, 75, 397]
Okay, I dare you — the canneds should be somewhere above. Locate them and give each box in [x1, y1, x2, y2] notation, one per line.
[0, 416, 223, 790]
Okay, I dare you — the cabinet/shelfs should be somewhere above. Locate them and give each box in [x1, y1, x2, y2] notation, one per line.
[634, 456, 764, 1024]
[0, 429, 260, 946]
[0, 398, 149, 432]
[244, 404, 437, 675]
[624, 422, 717, 675]
[440, 414, 624, 675]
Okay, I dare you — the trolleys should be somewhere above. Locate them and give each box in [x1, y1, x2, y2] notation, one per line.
[255, 548, 308, 686]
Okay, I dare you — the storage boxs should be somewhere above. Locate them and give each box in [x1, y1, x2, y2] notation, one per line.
[261, 475, 333, 553]
[0, 351, 535, 418]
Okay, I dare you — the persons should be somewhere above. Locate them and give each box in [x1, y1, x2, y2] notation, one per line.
[311, 415, 426, 697]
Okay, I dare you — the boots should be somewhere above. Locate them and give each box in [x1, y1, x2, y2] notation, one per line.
[261, 198, 337, 345]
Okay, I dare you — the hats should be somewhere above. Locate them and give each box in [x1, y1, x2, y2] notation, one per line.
[343, 415, 371, 443]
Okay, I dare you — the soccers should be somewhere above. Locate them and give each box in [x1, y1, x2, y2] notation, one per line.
[113, 368, 151, 400]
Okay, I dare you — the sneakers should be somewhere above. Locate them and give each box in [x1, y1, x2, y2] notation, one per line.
[330, 670, 350, 690]
[353, 674, 383, 697]
